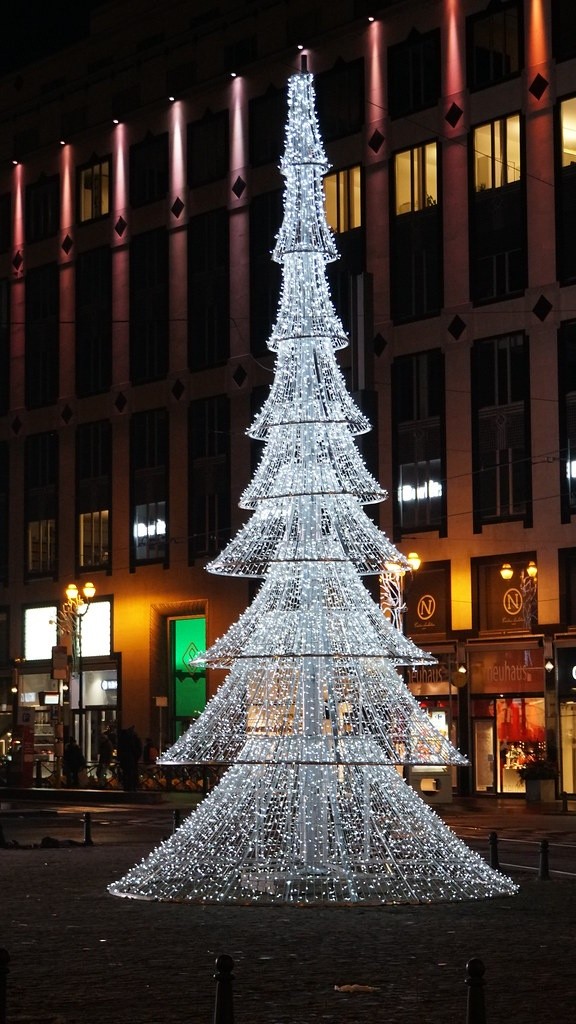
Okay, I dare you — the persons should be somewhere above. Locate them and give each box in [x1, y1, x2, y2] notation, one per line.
[61, 727, 157, 789]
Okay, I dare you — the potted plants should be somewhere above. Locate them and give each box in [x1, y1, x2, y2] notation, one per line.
[517, 760, 560, 802]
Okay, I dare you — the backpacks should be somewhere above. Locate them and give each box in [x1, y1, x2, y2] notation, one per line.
[149, 748, 158, 761]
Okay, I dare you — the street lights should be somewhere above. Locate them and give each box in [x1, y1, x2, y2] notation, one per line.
[386, 547, 423, 783]
[63, 581, 99, 753]
